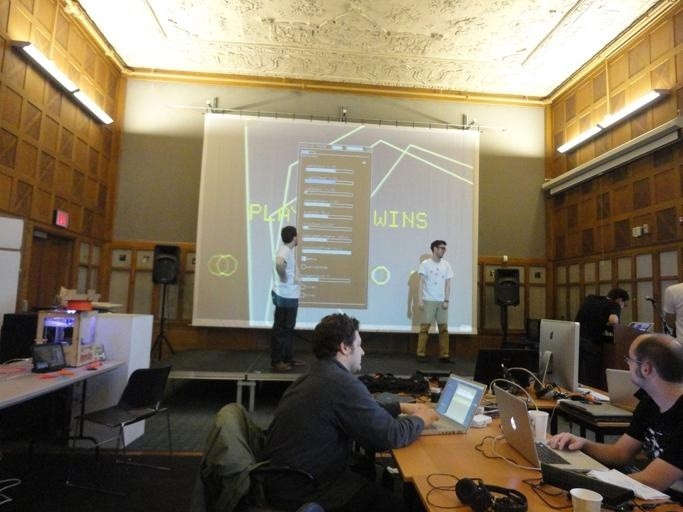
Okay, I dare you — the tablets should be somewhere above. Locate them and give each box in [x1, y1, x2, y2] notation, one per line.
[32, 342, 66, 373]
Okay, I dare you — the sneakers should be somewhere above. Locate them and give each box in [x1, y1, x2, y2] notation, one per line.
[270, 363, 292, 372]
[418, 353, 428, 361]
[440, 355, 451, 362]
[285, 357, 304, 365]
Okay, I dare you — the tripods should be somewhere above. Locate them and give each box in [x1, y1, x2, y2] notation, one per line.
[151, 285, 176, 360]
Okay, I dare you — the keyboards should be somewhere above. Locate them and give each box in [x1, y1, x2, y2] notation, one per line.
[577, 388, 609, 401]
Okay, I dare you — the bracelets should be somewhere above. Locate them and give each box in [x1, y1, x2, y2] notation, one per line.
[444, 299, 449, 303]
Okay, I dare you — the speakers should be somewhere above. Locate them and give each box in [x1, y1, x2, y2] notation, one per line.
[494, 269, 520, 306]
[152, 245, 180, 284]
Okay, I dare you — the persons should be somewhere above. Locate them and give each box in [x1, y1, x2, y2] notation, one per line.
[417, 240, 455, 364]
[264, 313, 440, 512]
[546, 334, 683, 506]
[575, 288, 629, 392]
[271, 226, 305, 372]
[663, 282, 683, 347]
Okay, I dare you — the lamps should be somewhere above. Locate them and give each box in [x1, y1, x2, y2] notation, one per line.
[599, 88, 670, 130]
[71, 88, 114, 125]
[14, 41, 75, 91]
[556, 125, 601, 154]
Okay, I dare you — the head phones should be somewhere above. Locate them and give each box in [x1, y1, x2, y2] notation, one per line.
[455, 478, 528, 512]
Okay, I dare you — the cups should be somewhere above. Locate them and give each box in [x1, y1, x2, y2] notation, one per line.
[528, 410, 549, 442]
[570, 488, 603, 512]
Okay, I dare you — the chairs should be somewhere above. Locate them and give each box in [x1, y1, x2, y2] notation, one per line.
[65, 365, 173, 496]
[203, 403, 322, 512]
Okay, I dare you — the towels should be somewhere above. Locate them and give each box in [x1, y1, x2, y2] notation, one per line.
[588, 468, 670, 500]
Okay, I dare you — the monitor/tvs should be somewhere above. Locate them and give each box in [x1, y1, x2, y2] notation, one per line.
[627, 322, 654, 332]
[533, 319, 579, 400]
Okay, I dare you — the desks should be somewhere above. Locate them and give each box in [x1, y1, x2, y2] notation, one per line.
[0, 361, 125, 437]
[507, 374, 635, 445]
[410, 476, 683, 512]
[393, 403, 610, 480]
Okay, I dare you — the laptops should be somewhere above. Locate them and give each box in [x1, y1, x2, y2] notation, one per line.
[558, 399, 634, 423]
[492, 383, 612, 473]
[416, 374, 487, 435]
[606, 368, 640, 412]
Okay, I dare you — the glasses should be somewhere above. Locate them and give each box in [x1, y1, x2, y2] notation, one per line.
[622, 353, 645, 365]
[436, 246, 445, 249]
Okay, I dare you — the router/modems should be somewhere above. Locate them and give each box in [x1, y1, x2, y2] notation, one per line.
[472, 413, 492, 426]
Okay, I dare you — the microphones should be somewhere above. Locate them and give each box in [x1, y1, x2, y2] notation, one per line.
[644, 296, 656, 302]
